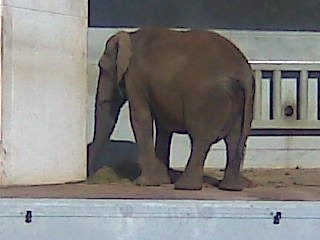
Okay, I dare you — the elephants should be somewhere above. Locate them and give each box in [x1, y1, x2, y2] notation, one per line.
[87, 24, 255, 190]
